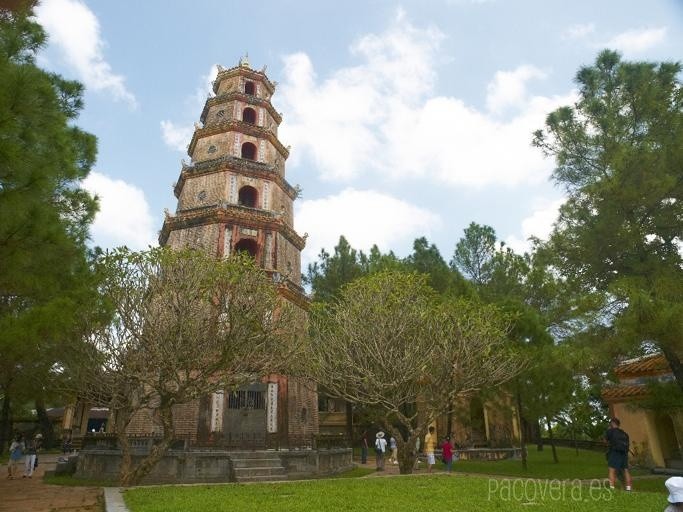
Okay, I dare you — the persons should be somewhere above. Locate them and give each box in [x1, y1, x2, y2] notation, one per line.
[423, 427, 439, 472]
[660, 474, 683, 512]
[359, 428, 370, 464]
[22, 433, 41, 478]
[388, 436, 399, 464]
[4, 433, 25, 478]
[602, 416, 633, 492]
[374, 430, 388, 472]
[441, 433, 457, 472]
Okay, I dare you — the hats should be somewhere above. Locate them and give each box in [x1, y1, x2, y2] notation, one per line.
[376, 431, 385, 438]
[664, 476, 682, 503]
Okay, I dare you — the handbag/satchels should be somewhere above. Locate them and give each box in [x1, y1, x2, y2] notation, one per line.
[375, 439, 382, 453]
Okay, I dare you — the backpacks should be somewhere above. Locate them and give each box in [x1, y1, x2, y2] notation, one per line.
[609, 430, 629, 452]
